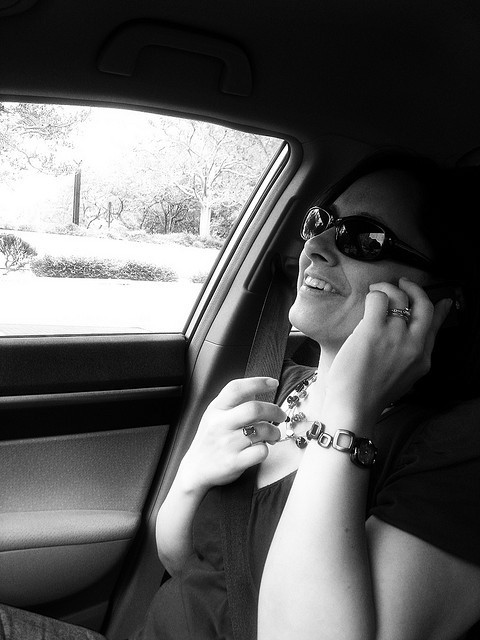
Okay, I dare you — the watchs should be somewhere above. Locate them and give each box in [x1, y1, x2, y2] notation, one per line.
[309, 420, 378, 469]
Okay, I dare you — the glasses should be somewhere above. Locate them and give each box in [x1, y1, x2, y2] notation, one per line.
[299, 205, 457, 275]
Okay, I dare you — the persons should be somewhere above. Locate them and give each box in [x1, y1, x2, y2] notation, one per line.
[0, 151, 480, 640]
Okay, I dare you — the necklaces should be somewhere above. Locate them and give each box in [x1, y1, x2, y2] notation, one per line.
[287, 371, 321, 448]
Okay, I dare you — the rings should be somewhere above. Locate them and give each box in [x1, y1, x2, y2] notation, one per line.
[387, 307, 410, 320]
[242, 425, 256, 443]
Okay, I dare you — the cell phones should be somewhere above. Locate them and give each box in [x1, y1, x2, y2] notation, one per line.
[405, 284, 471, 328]
